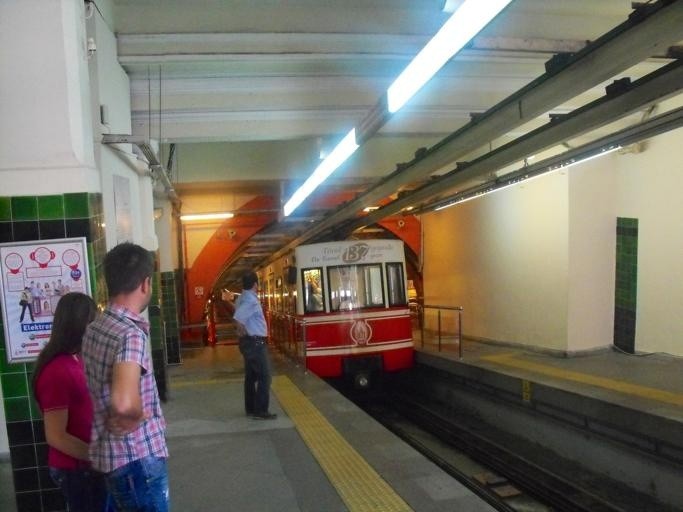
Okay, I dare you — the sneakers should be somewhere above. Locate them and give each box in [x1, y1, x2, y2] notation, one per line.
[246, 411, 253, 415]
[251, 414, 278, 420]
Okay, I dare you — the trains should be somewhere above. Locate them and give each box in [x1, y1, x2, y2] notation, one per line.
[218, 239, 417, 388]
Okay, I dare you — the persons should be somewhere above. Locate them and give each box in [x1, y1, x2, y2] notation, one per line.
[33, 290, 102, 511]
[232, 271, 277, 419]
[308, 284, 321, 314]
[81, 242, 172, 511]
[18, 279, 72, 322]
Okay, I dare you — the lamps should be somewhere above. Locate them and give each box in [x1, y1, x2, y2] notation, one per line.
[283, 0, 512, 218]
